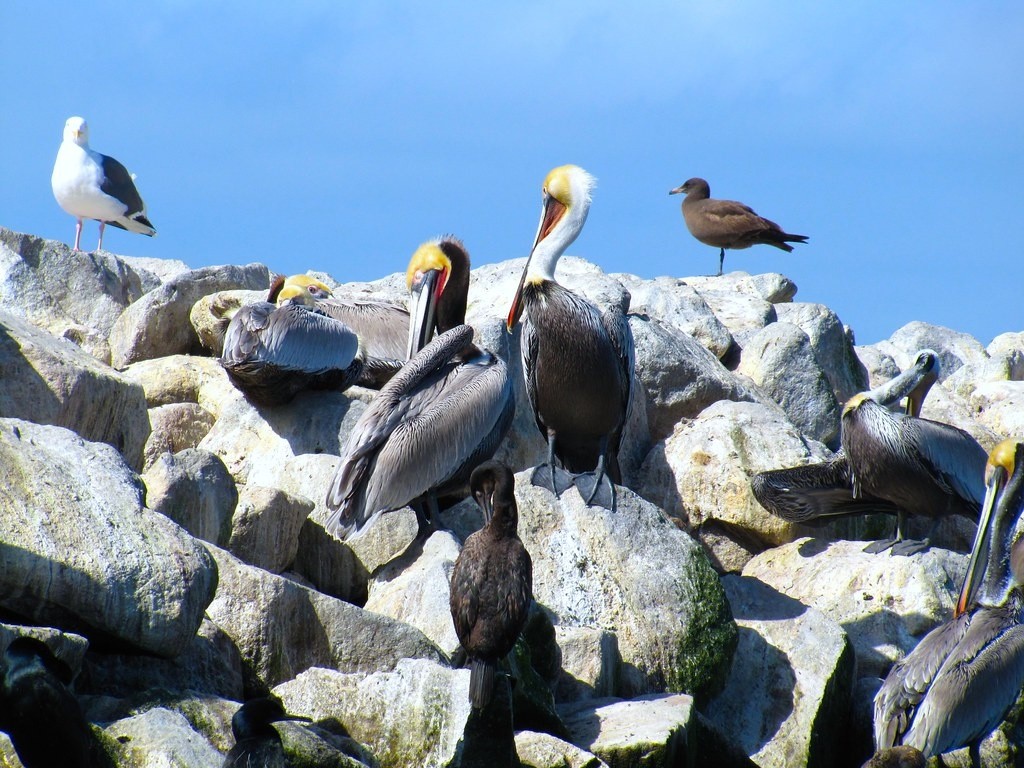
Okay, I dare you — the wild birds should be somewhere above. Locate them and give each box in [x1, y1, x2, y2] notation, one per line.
[324, 237, 516, 545]
[669, 176, 810, 279]
[508, 164, 634, 512]
[51, 116, 155, 257]
[219, 270, 367, 404]
[868, 441, 1023, 768]
[449, 460, 534, 708]
[750, 348, 992, 555]
